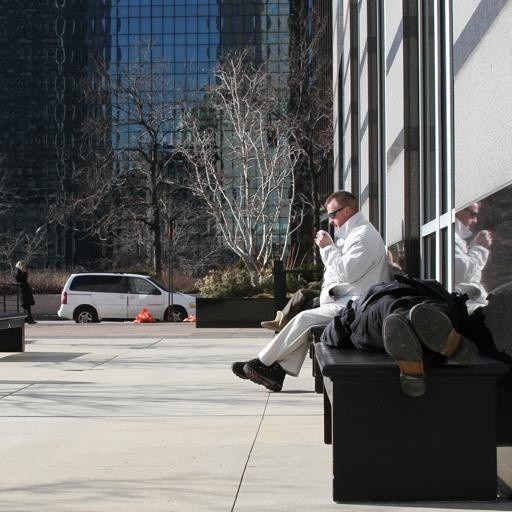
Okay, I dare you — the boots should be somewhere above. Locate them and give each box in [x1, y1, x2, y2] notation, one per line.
[259, 311, 289, 332]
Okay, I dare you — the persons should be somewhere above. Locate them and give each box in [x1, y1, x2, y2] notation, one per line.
[230, 190, 390, 392]
[261, 289, 321, 335]
[454, 203, 493, 314]
[16, 260, 38, 324]
[321, 274, 498, 397]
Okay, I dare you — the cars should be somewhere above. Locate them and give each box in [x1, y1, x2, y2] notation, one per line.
[56, 269, 197, 324]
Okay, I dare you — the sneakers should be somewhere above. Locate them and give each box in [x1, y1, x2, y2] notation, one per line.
[230, 357, 288, 393]
[409, 300, 480, 365]
[25, 320, 37, 324]
[381, 312, 429, 398]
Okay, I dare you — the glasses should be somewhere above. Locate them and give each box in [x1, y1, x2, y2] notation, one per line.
[328, 206, 347, 219]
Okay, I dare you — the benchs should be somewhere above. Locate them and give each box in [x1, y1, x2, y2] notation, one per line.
[310, 327, 512, 502]
